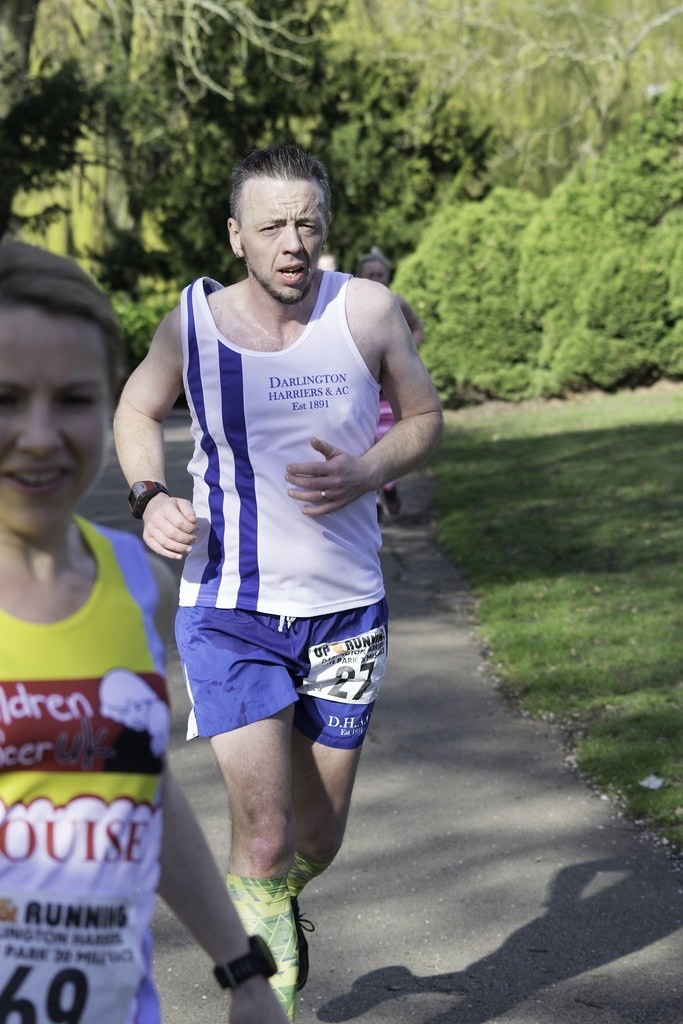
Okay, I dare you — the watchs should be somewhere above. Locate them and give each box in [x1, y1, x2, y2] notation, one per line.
[128, 481, 170, 520]
[213, 935, 277, 993]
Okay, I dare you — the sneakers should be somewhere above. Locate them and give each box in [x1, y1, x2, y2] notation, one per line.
[289, 893, 314, 992]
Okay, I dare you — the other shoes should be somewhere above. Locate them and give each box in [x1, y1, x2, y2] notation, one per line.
[376, 504, 382, 522]
[386, 491, 400, 513]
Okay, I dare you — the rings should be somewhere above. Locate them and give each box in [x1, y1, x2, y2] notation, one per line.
[321, 490, 326, 499]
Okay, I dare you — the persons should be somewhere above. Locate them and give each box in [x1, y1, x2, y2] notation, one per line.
[352, 256, 423, 520]
[0, 240, 293, 1024]
[114, 144, 441, 1024]
